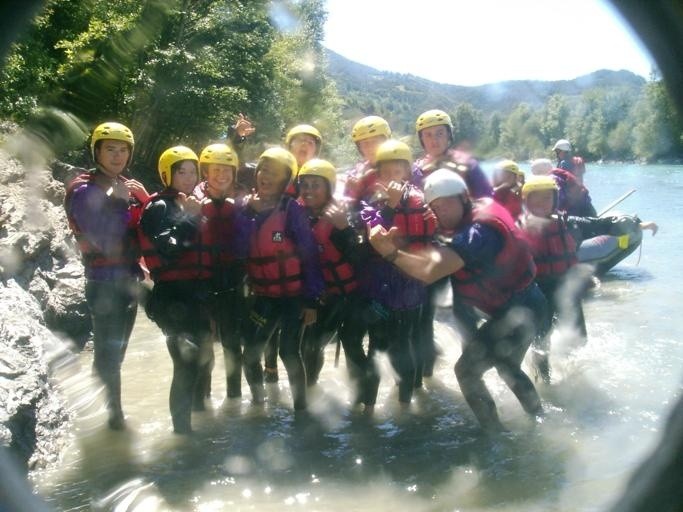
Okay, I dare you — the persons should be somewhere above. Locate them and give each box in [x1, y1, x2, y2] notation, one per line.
[64, 122, 152, 434]
[138, 109, 658, 432]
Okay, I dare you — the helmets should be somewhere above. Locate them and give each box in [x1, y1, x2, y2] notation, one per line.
[352, 116, 411, 175]
[158, 143, 239, 187]
[255, 125, 336, 197]
[416, 109, 453, 146]
[90, 122, 135, 166]
[495, 158, 558, 202]
[424, 168, 468, 204]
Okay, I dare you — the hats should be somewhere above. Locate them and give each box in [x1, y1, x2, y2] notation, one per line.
[552, 139, 571, 151]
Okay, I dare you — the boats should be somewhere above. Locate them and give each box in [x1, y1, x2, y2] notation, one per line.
[580, 229, 642, 279]
